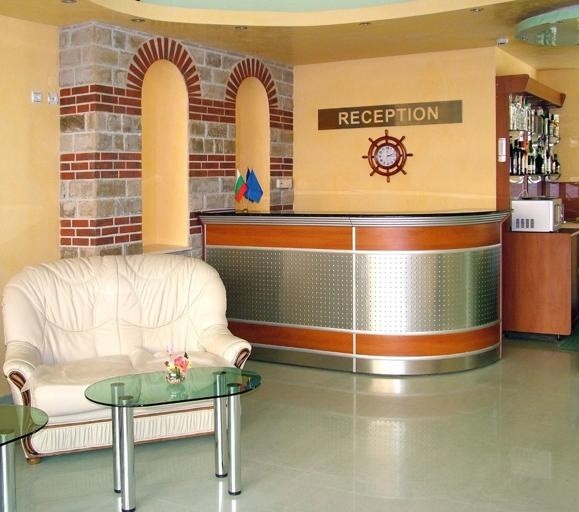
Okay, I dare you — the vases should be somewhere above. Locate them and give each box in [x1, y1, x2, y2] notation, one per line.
[166, 372, 185, 384]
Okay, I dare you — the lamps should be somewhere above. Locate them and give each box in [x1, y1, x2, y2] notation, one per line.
[498, 136, 507, 162]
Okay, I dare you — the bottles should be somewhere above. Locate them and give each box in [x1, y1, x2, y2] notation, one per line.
[509, 95, 561, 175]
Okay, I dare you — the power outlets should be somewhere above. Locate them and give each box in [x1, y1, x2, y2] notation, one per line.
[276, 178, 292, 188]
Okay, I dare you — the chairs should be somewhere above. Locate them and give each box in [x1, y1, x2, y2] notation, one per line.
[2, 253, 252, 464]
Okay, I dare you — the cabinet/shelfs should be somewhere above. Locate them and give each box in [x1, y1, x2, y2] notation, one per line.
[496, 74, 579, 341]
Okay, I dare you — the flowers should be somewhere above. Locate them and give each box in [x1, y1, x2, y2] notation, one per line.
[163, 344, 192, 379]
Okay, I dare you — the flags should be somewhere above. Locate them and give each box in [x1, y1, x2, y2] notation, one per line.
[245, 170, 263, 203]
[233, 168, 247, 203]
[243, 167, 254, 203]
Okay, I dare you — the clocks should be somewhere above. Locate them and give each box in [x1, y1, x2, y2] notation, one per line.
[362, 130, 413, 183]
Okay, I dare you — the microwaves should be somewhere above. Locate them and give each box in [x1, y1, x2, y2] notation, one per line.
[511, 196, 563, 233]
